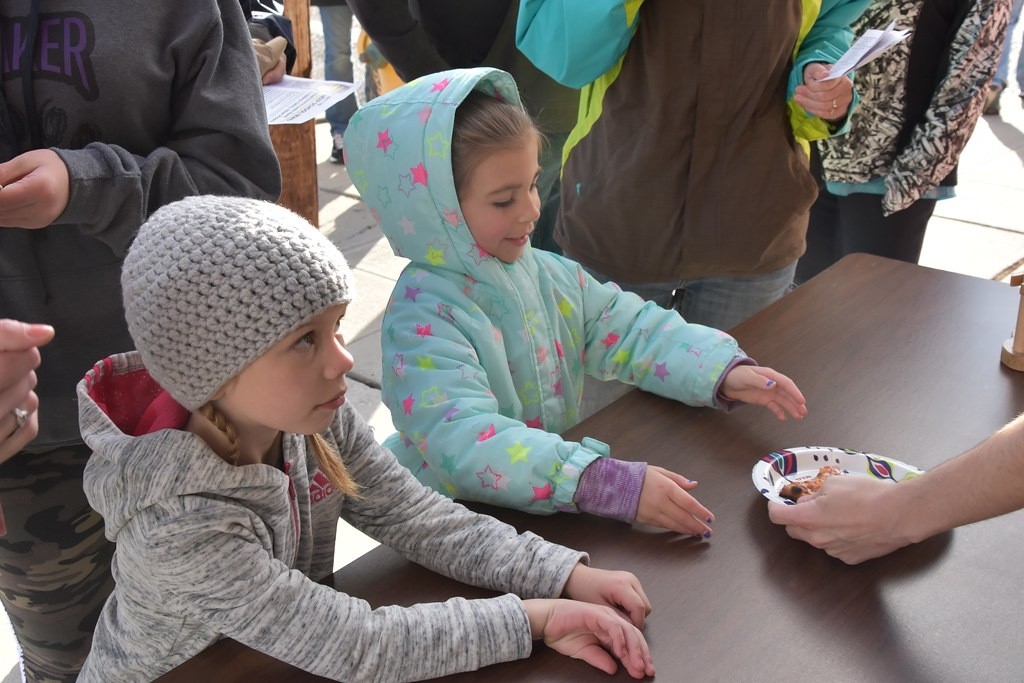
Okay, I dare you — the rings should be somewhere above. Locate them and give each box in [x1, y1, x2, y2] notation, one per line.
[831, 98, 837, 108]
[8, 407, 30, 429]
[829, 110, 835, 114]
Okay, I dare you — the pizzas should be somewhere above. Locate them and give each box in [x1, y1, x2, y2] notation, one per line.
[779, 465, 838, 502]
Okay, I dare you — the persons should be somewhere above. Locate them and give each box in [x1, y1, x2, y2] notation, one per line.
[247, 0, 1024, 565]
[73, 194, 656, 683]
[0, 0, 285, 683]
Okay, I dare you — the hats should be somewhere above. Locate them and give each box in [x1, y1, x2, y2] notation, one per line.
[120, 195, 355, 413]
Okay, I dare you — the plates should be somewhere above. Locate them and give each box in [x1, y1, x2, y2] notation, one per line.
[751, 447, 924, 506]
